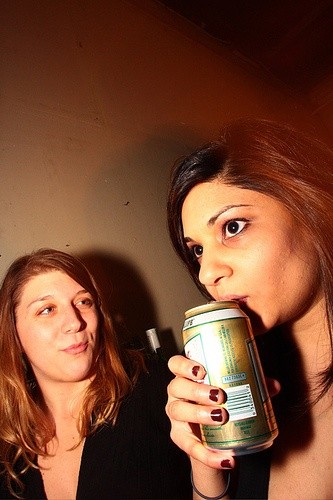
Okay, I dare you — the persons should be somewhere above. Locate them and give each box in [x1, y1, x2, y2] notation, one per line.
[0, 246, 196, 500]
[160, 118, 333, 500]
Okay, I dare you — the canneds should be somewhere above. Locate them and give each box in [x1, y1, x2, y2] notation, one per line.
[181, 300, 278, 456]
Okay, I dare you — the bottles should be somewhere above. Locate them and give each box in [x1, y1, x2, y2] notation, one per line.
[145, 327, 169, 374]
